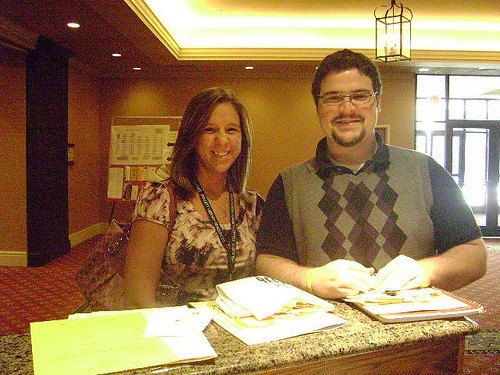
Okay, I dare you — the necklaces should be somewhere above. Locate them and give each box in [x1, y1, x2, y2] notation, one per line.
[203, 189, 229, 218]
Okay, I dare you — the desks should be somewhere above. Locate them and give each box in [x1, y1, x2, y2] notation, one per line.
[0, 265, 483, 375]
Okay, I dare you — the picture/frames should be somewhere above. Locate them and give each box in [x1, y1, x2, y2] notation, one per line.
[375, 123, 391, 145]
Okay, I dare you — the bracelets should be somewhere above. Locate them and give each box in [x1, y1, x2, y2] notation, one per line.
[307, 267, 317, 293]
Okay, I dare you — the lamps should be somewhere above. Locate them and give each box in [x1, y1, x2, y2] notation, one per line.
[372, 0, 414, 63]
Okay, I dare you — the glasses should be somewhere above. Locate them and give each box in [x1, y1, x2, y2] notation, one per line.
[317, 91, 378, 106]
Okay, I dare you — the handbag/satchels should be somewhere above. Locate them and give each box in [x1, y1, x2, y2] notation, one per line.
[75, 182, 180, 311]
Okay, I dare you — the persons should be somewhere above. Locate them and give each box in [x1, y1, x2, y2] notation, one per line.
[124, 88, 265, 310]
[256, 48, 488, 292]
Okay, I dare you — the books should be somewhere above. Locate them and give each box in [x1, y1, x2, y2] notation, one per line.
[353, 285, 487, 323]
